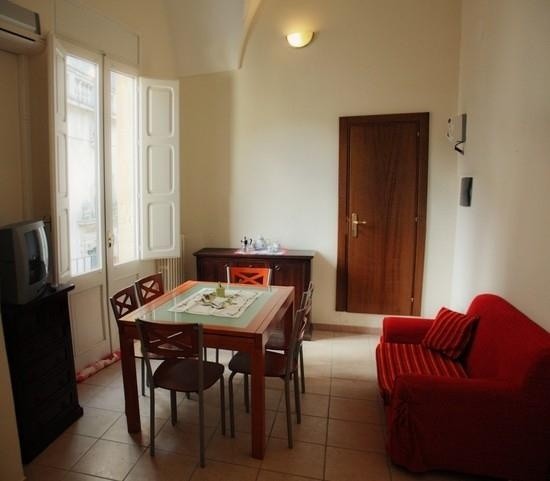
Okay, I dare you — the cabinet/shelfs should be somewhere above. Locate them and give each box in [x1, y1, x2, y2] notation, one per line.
[1, 283, 84, 465]
[193, 248, 314, 341]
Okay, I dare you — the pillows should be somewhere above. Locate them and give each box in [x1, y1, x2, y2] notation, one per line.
[422, 307, 479, 360]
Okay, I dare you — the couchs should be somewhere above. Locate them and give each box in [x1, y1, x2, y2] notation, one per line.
[376, 294, 549, 481]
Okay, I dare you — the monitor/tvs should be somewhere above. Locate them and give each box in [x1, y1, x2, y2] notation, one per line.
[0, 219, 52, 309]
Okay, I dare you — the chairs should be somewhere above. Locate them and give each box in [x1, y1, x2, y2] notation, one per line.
[216, 266, 272, 364]
[135, 272, 207, 361]
[109, 285, 192, 399]
[266, 281, 315, 393]
[229, 303, 313, 448]
[135, 319, 225, 468]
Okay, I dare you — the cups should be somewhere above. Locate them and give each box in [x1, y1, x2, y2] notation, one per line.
[271, 242, 280, 251]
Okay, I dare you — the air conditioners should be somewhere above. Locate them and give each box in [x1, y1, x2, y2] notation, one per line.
[0, 0, 47, 54]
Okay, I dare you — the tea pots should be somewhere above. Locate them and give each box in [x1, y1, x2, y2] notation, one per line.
[239, 236, 253, 251]
[253, 234, 271, 251]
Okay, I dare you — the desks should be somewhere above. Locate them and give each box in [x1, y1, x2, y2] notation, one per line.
[117, 279, 296, 460]
